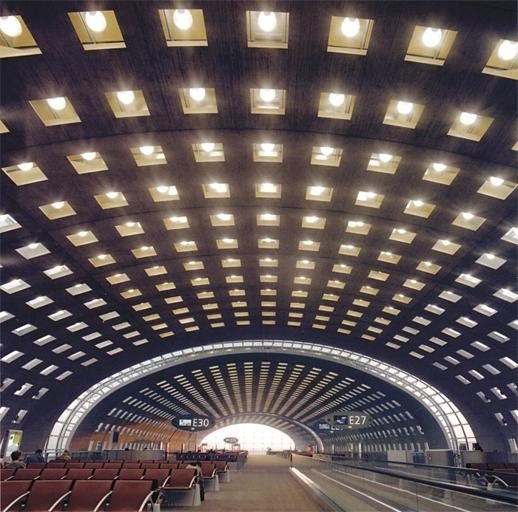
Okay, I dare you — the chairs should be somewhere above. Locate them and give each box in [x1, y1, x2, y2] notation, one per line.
[0, 449, 248, 512]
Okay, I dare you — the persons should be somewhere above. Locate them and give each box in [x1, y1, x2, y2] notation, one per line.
[185, 462, 205, 501]
[0, 449, 71, 470]
